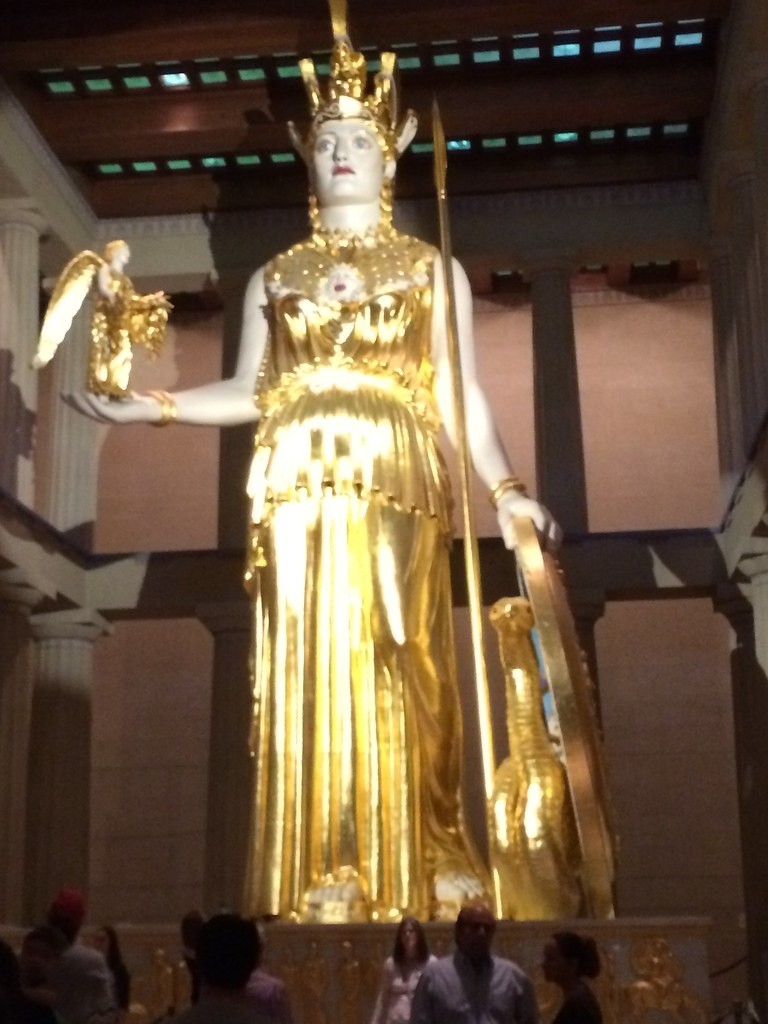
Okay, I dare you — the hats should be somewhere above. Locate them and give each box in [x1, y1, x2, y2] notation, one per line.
[56, 890, 88, 918]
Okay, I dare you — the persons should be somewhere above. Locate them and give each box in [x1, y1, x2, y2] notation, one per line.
[370, 918, 439, 1024]
[409, 908, 537, 1024]
[542, 930, 603, 1024]
[87, 240, 174, 400]
[0, 890, 130, 1024]
[62, 0, 559, 924]
[180, 910, 293, 1024]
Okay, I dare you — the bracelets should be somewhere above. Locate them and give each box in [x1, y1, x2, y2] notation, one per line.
[148, 390, 177, 427]
[489, 477, 524, 507]
